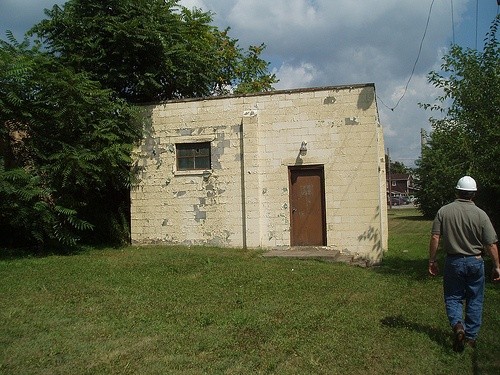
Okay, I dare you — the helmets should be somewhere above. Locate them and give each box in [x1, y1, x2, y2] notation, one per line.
[455, 176, 477, 191]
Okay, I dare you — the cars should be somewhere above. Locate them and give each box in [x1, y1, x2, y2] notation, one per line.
[388, 192, 409, 206]
[409, 195, 418, 202]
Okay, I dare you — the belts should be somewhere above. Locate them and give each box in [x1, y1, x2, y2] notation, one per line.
[449, 252, 482, 257]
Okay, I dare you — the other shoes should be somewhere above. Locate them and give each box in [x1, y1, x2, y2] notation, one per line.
[468, 340, 476, 347]
[453, 325, 465, 352]
